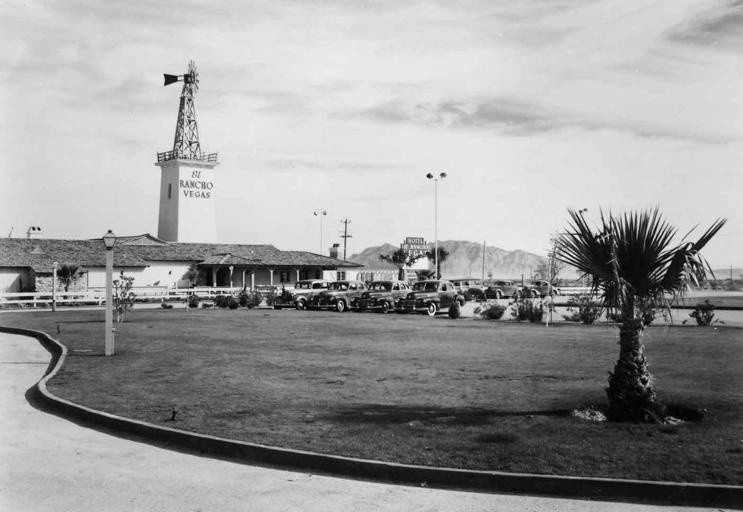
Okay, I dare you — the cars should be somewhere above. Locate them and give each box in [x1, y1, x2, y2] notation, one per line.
[271, 278, 562, 319]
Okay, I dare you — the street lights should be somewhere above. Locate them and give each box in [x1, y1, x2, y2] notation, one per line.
[103, 228, 118, 358]
[314, 209, 327, 257]
[425, 170, 448, 280]
[52, 260, 59, 312]
[578, 207, 589, 288]
